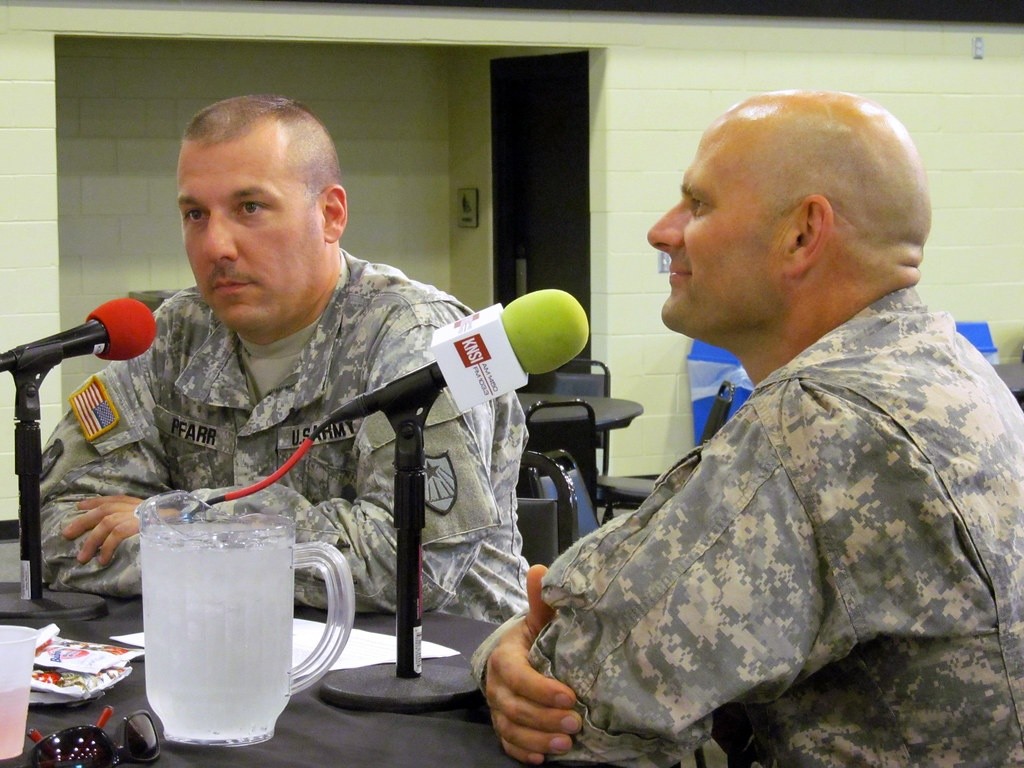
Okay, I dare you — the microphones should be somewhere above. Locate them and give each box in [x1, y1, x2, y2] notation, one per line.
[0, 297, 156, 372]
[329, 290, 589, 425]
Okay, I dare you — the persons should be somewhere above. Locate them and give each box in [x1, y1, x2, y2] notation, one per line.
[39, 95, 530, 625]
[473, 90, 1024, 768]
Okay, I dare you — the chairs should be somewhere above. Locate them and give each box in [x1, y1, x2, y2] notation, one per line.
[518, 359, 613, 484]
[517, 451, 576, 568]
[600, 381, 734, 527]
[526, 401, 598, 516]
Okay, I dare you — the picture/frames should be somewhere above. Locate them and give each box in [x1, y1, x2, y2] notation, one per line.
[457, 188, 478, 228]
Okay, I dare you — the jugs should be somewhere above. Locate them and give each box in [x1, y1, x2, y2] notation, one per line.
[134, 488, 354, 746]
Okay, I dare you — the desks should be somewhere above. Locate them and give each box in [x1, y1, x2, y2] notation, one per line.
[0, 581, 679, 768]
[514, 395, 642, 531]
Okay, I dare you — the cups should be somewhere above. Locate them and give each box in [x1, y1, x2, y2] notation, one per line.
[0, 625, 41, 760]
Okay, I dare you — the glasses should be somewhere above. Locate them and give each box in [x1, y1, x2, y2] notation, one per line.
[26, 706, 160, 768]
